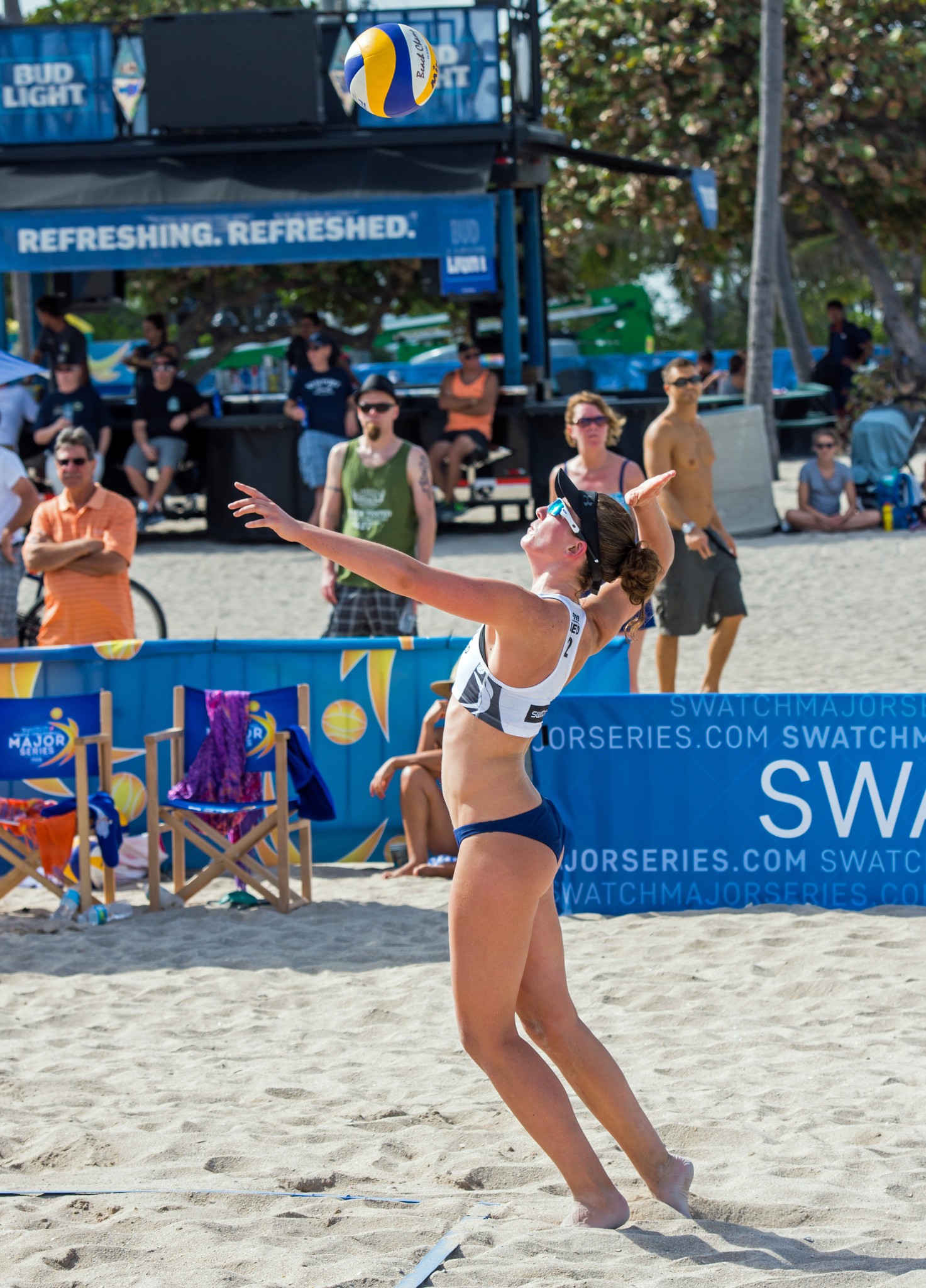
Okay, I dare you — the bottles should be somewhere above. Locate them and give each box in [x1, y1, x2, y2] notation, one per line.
[212, 392, 223, 419]
[53, 880, 80, 920]
[62, 401, 74, 422]
[137, 882, 185, 909]
[882, 502, 894, 532]
[76, 899, 133, 925]
[219, 357, 291, 394]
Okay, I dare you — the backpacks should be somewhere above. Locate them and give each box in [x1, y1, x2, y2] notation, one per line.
[878, 474, 914, 528]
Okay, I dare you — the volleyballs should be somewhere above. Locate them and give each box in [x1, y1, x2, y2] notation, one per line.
[62, 834, 105, 890]
[343, 23, 439, 119]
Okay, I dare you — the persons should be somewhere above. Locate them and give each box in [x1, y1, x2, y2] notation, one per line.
[226, 471, 692, 1233]
[0, 290, 926, 880]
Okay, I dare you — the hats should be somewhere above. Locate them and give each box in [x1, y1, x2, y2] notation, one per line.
[307, 333, 331, 350]
[554, 468, 602, 596]
[53, 339, 87, 364]
[458, 339, 478, 354]
[352, 374, 403, 404]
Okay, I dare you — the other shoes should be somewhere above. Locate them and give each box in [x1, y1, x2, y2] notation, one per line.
[435, 500, 465, 524]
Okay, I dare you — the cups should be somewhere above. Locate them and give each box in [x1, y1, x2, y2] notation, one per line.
[389, 843, 408, 868]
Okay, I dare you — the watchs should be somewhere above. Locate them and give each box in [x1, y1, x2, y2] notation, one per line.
[681, 522, 696, 534]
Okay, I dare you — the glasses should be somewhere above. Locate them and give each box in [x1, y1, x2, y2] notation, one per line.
[673, 375, 701, 387]
[817, 443, 834, 449]
[56, 368, 72, 372]
[547, 497, 599, 563]
[464, 352, 479, 360]
[356, 401, 394, 415]
[57, 457, 86, 467]
[569, 416, 608, 427]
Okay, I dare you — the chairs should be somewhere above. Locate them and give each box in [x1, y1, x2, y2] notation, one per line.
[0, 691, 116, 920]
[144, 686, 311, 912]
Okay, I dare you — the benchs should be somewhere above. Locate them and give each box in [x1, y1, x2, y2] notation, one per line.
[23, 387, 532, 528]
